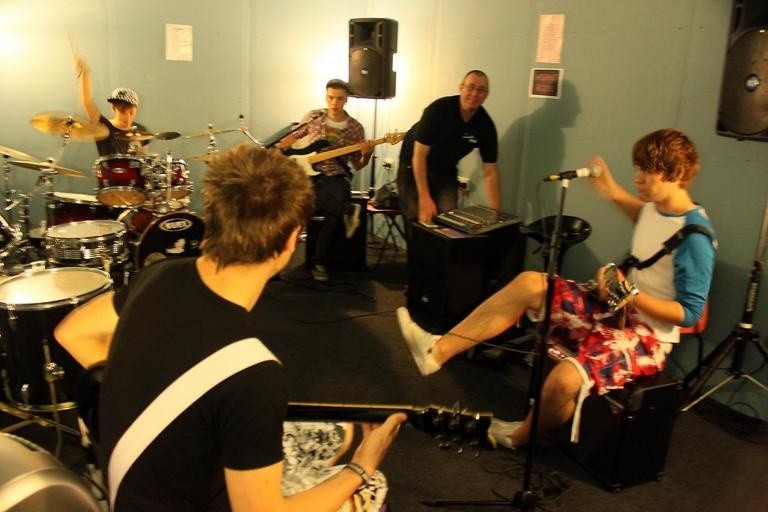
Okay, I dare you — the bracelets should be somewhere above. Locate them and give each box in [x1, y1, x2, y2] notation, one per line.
[342, 462, 370, 491]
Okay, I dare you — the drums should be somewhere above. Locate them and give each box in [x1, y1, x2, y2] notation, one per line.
[0, 265, 118, 414]
[47, 220, 131, 272]
[93, 152, 149, 208]
[147, 157, 193, 203]
[45, 191, 118, 228]
[118, 204, 206, 281]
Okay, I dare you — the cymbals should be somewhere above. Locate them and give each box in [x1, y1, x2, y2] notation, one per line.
[184, 128, 241, 139]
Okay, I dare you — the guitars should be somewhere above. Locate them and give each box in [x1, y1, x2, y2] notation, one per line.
[113, 131, 154, 143]
[587, 260, 643, 317]
[0, 145, 36, 160]
[280, 131, 409, 185]
[73, 362, 495, 465]
[30, 111, 110, 141]
[10, 155, 85, 180]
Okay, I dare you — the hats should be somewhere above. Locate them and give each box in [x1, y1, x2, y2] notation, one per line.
[108, 89, 137, 104]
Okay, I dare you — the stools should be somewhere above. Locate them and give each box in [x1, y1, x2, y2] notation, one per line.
[363, 195, 413, 268]
[480, 216, 591, 373]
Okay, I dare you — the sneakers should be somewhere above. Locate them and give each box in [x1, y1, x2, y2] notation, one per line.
[490, 418, 524, 450]
[312, 265, 329, 281]
[397, 307, 441, 377]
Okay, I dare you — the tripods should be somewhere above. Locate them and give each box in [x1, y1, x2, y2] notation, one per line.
[356, 100, 400, 252]
[417, 247, 557, 512]
[682, 252, 768, 418]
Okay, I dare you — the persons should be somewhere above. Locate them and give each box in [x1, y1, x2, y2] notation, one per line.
[396, 128, 719, 449]
[275, 79, 375, 281]
[396, 70, 502, 224]
[50, 146, 409, 512]
[77, 55, 150, 157]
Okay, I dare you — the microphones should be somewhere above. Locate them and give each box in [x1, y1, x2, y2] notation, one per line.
[541, 163, 605, 188]
[305, 110, 327, 127]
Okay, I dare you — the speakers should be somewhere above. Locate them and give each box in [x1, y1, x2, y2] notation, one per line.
[348, 18, 398, 99]
[713, 0, 768, 143]
[305, 213, 368, 284]
